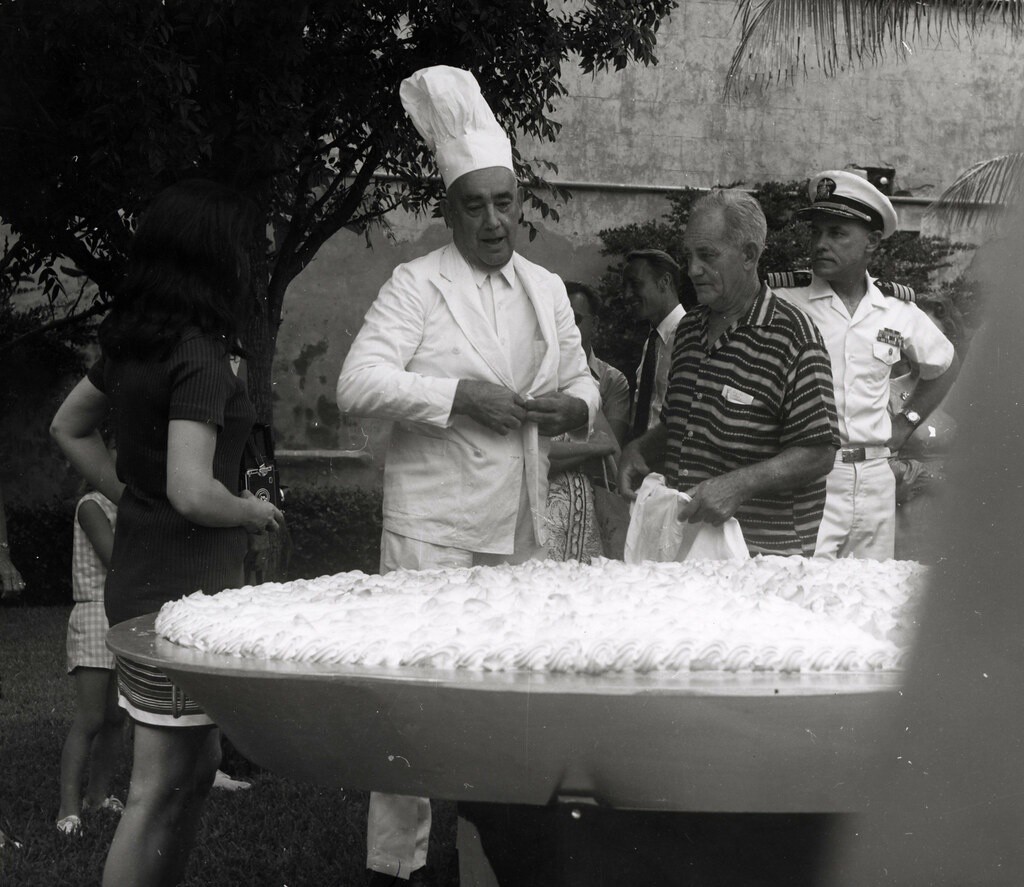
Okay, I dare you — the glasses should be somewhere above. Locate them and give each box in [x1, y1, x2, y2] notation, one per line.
[574, 312, 596, 326]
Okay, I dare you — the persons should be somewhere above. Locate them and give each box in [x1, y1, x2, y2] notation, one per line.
[616, 188, 841, 563]
[337, 167, 602, 887]
[46, 187, 286, 886]
[543, 281, 628, 566]
[762, 170, 963, 563]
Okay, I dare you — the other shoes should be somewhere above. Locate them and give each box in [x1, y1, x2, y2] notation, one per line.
[212, 768, 252, 793]
[54, 812, 83, 837]
[80, 795, 125, 817]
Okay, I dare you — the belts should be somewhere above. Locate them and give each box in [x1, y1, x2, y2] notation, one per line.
[835, 447, 892, 463]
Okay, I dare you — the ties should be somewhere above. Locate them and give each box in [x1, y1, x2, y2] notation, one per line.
[633, 328, 659, 441]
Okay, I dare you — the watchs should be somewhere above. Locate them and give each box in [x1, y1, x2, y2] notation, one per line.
[899, 408, 921, 426]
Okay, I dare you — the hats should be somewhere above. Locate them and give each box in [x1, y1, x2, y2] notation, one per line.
[399, 65, 514, 191]
[794, 170, 897, 239]
